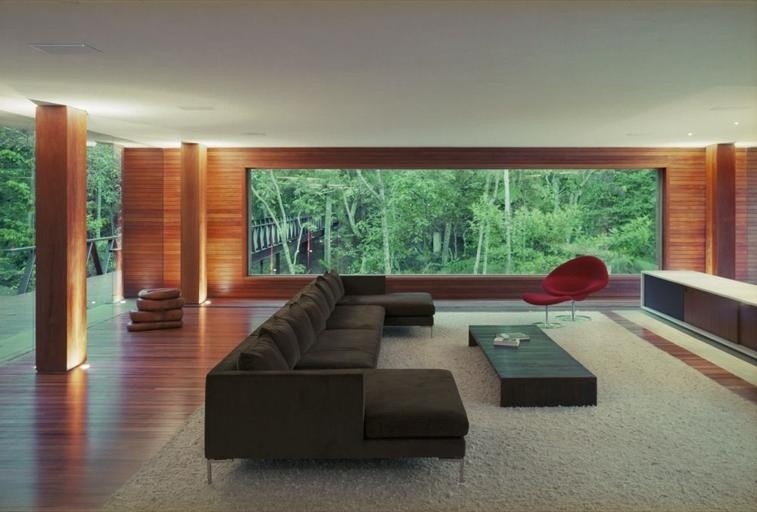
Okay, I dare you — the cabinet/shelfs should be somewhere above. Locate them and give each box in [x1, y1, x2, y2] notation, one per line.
[640, 268, 757, 359]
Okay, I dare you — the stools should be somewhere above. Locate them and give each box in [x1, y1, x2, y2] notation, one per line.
[522, 295, 572, 326]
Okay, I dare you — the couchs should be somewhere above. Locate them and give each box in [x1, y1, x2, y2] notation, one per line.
[201, 270, 468, 485]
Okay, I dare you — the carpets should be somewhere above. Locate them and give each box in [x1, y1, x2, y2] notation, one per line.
[97, 311, 754, 511]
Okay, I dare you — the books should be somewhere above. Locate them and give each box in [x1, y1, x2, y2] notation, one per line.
[493, 332, 530, 346]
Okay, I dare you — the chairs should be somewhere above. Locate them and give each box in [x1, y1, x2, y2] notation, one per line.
[543, 256, 606, 327]
